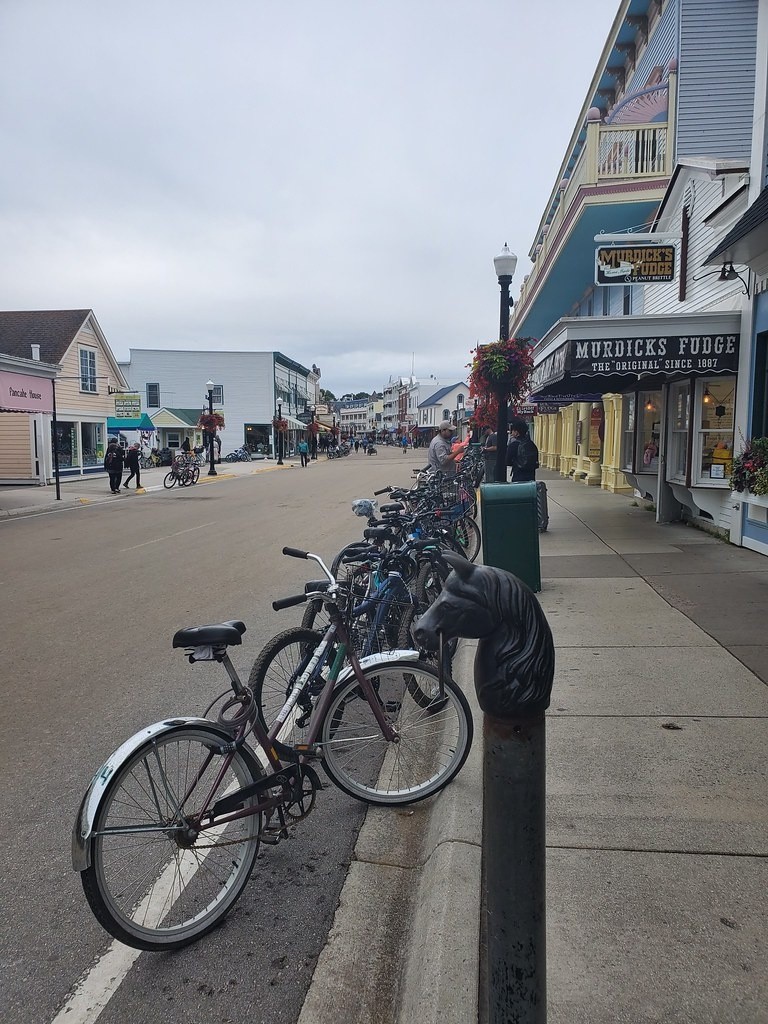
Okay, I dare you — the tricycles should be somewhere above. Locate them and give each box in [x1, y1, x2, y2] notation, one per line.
[366, 444, 378, 456]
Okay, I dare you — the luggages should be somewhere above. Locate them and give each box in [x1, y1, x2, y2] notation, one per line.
[535, 479, 549, 534]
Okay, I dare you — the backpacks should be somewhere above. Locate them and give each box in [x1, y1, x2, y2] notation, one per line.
[514, 439, 539, 472]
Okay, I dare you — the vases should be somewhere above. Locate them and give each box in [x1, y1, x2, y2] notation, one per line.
[498, 365, 516, 384]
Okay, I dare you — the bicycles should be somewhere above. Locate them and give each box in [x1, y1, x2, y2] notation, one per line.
[224, 438, 487, 763]
[402, 443, 407, 455]
[137, 446, 207, 489]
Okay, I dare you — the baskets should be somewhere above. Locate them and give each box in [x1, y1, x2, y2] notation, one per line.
[344, 593, 413, 655]
[429, 483, 475, 521]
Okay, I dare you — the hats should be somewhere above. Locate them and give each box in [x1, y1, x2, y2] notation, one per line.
[439, 420, 458, 430]
[482, 425, 490, 432]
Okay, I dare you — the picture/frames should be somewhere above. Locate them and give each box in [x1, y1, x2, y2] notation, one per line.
[710, 463, 725, 479]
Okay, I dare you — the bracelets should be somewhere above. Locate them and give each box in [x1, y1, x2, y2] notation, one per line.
[485, 448, 486, 451]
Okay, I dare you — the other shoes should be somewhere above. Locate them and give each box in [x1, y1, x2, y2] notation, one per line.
[137, 486, 143, 488]
[111, 491, 116, 494]
[123, 483, 129, 488]
[116, 490, 120, 493]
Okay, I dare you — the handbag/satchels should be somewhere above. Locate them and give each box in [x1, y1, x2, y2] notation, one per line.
[307, 455, 310, 462]
[124, 458, 129, 468]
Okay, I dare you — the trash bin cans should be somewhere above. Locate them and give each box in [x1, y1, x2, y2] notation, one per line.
[478, 481, 543, 593]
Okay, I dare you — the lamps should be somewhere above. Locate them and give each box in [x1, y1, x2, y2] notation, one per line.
[718, 261, 738, 281]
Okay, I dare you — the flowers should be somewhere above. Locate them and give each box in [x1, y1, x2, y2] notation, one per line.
[726, 436, 768, 496]
[468, 338, 537, 425]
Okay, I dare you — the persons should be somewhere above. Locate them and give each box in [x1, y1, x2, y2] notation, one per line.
[427, 420, 465, 471]
[507, 418, 539, 481]
[351, 437, 368, 454]
[104, 438, 125, 494]
[451, 429, 470, 461]
[181, 437, 190, 457]
[402, 436, 408, 454]
[481, 425, 497, 482]
[299, 440, 309, 468]
[123, 443, 143, 488]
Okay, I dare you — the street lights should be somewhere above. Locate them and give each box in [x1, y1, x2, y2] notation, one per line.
[397, 420, 401, 441]
[331, 412, 337, 440]
[310, 405, 316, 459]
[70, 547, 475, 955]
[337, 418, 340, 439]
[206, 379, 218, 475]
[414, 419, 419, 447]
[276, 397, 284, 465]
[490, 242, 519, 482]
